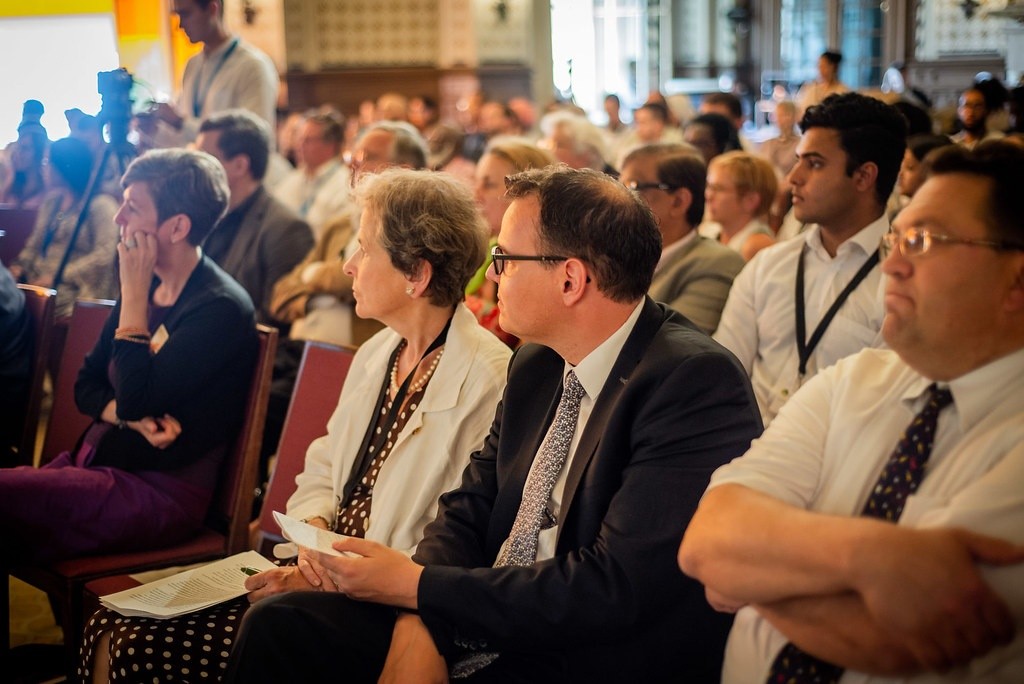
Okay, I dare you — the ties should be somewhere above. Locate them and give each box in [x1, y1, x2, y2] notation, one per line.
[495, 369, 587, 567]
[764, 383, 954, 684]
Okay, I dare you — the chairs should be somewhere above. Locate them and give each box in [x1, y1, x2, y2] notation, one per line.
[0, 202, 359, 684]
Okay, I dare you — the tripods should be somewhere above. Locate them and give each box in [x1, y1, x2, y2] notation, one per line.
[51, 99, 141, 288]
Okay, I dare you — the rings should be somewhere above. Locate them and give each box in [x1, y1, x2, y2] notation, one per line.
[126, 240, 136, 248]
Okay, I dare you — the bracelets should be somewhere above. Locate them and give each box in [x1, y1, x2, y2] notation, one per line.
[115, 328, 150, 344]
[119, 420, 128, 430]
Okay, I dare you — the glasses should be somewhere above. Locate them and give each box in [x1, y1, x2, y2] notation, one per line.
[343, 149, 388, 168]
[492, 245, 592, 283]
[880, 228, 1003, 259]
[627, 182, 671, 193]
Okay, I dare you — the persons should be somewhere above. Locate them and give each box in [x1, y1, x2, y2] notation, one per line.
[0, 0, 1024, 684]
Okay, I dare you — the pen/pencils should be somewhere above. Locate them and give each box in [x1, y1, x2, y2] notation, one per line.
[241, 567, 261, 575]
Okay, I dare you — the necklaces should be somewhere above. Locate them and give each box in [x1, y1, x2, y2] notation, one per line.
[191, 37, 239, 118]
[391, 339, 445, 394]
[40, 192, 74, 259]
